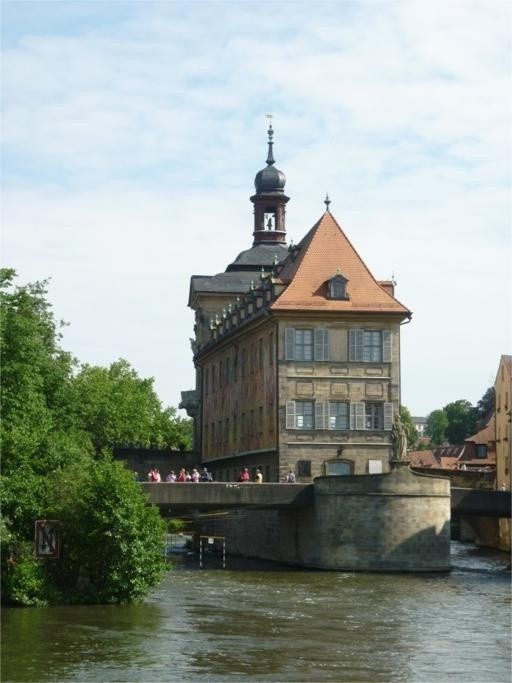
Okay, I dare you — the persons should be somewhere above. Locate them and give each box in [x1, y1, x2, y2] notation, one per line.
[237, 467, 249, 482]
[390, 410, 410, 460]
[147, 464, 215, 482]
[253, 467, 265, 483]
[283, 471, 288, 482]
[287, 469, 296, 481]
